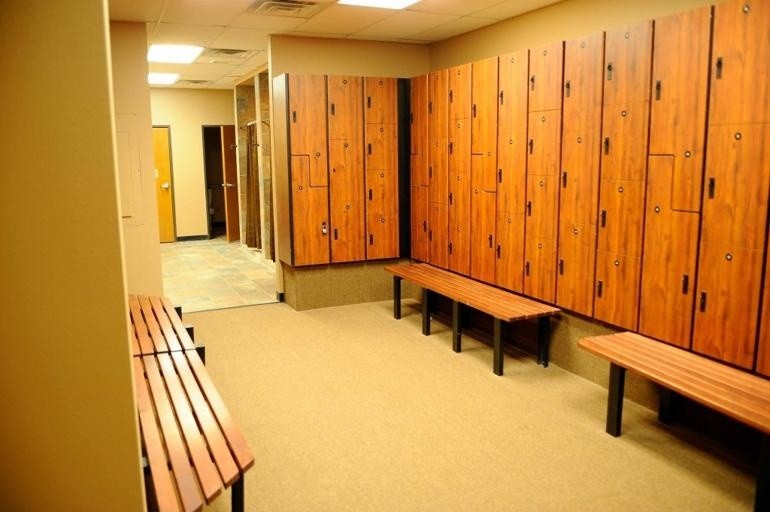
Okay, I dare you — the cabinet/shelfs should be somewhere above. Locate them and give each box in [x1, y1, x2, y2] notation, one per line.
[271, 71, 406, 268]
[408, 3, 767, 379]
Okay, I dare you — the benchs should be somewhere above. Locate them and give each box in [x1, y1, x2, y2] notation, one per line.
[127, 294, 255, 511]
[577, 331, 770, 510]
[383, 261, 562, 377]
